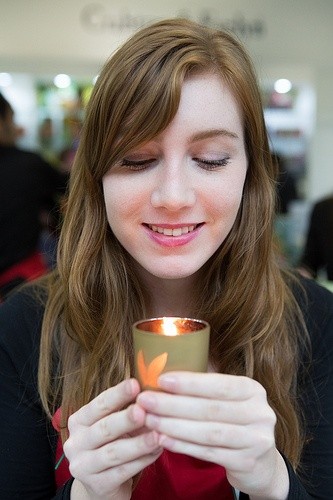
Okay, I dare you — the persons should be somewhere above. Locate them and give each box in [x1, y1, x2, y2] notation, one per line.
[296, 190, 332, 294]
[1, 18, 332, 500]
[0, 95, 73, 297]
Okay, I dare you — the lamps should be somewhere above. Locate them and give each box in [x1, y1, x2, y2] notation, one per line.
[131, 315, 210, 393]
